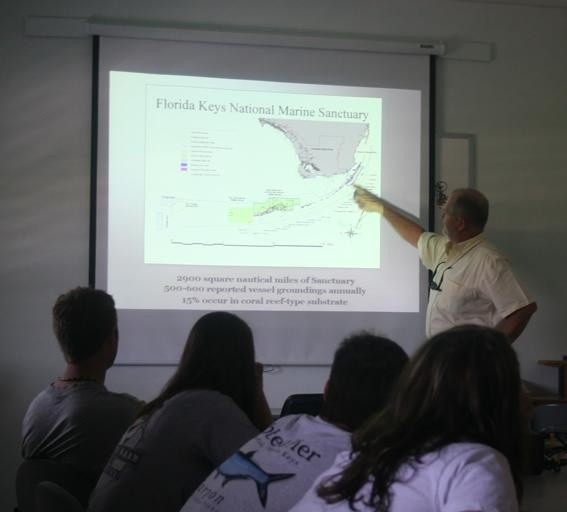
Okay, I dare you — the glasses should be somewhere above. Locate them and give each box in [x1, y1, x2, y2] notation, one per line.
[425, 261, 451, 294]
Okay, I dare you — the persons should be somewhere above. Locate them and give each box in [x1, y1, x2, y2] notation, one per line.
[352, 183, 537, 345]
[286, 322, 526, 512]
[19, 286, 151, 496]
[175, 330, 412, 512]
[79, 309, 275, 511]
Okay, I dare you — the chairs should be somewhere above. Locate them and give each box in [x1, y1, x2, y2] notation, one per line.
[15, 459, 92, 512]
[280, 394, 323, 417]
